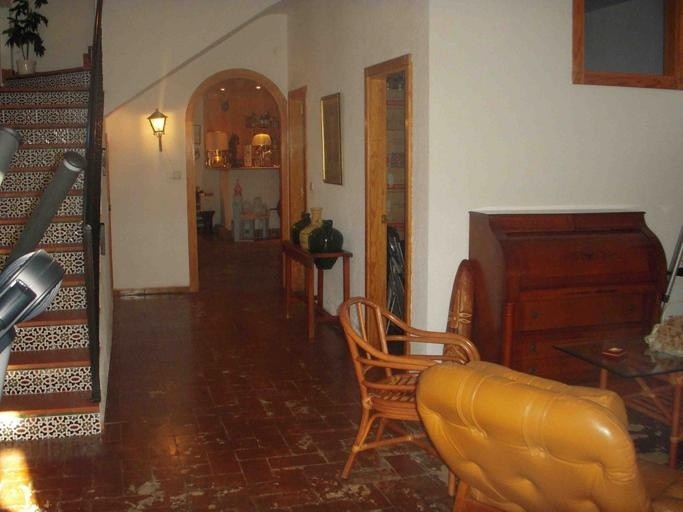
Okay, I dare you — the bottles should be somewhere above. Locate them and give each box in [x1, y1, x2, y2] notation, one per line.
[258, 111, 274, 128]
[198, 191, 205, 211]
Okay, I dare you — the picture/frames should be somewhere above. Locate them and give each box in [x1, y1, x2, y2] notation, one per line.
[319, 92, 344, 186]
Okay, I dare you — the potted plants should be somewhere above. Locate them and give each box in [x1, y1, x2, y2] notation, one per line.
[0, 0, 48, 74]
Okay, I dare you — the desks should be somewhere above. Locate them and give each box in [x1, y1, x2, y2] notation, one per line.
[279, 239, 352, 344]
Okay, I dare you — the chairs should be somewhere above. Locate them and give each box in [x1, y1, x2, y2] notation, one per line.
[336, 295, 482, 496]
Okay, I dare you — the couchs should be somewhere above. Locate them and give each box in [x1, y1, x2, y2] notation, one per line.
[414, 359, 682, 511]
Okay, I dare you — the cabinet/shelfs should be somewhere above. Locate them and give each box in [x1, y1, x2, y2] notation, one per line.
[466, 208, 668, 386]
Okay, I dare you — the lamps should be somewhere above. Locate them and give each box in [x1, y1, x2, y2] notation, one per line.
[251, 133, 272, 167]
[147, 108, 169, 152]
[205, 130, 229, 165]
